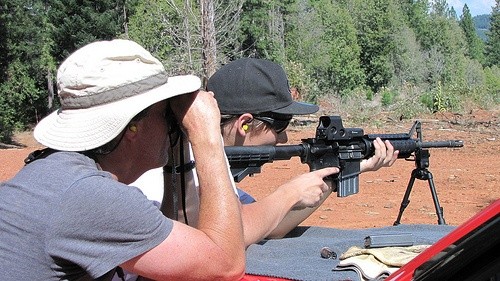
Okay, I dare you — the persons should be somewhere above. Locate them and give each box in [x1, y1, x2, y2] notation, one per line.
[0, 39, 246, 281]
[128, 58, 398, 250]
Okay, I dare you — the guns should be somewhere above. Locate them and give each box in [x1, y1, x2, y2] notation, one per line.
[223, 114, 464, 225]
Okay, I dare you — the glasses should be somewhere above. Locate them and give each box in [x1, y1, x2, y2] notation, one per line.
[254, 114, 292, 134]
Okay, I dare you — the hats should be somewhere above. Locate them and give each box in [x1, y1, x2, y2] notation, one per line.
[33, 39, 202, 151]
[206, 58, 319, 115]
